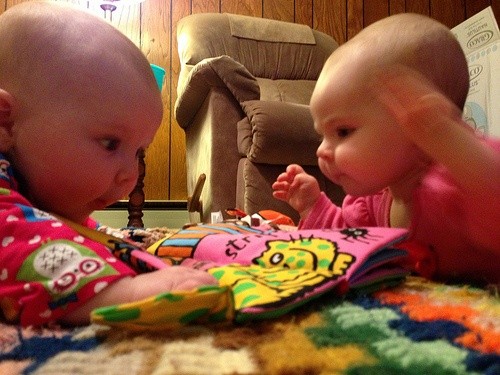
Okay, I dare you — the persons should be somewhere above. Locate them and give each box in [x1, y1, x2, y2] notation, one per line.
[273, 13, 500, 277]
[0, 2, 221, 322]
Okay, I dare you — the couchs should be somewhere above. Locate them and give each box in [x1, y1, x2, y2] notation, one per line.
[175, 13, 345, 225]
